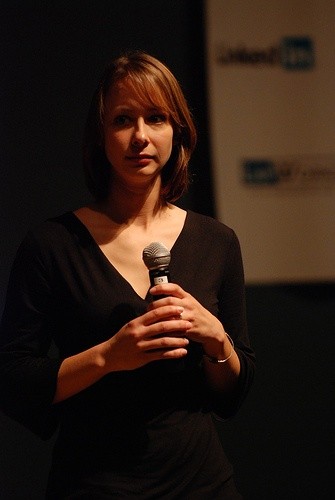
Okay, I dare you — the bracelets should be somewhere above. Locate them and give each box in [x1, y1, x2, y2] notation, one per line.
[204, 332, 237, 363]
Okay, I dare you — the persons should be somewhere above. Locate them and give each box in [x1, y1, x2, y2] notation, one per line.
[1, 52, 260, 500]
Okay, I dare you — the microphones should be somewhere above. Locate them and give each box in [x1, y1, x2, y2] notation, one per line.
[142, 241, 178, 362]
